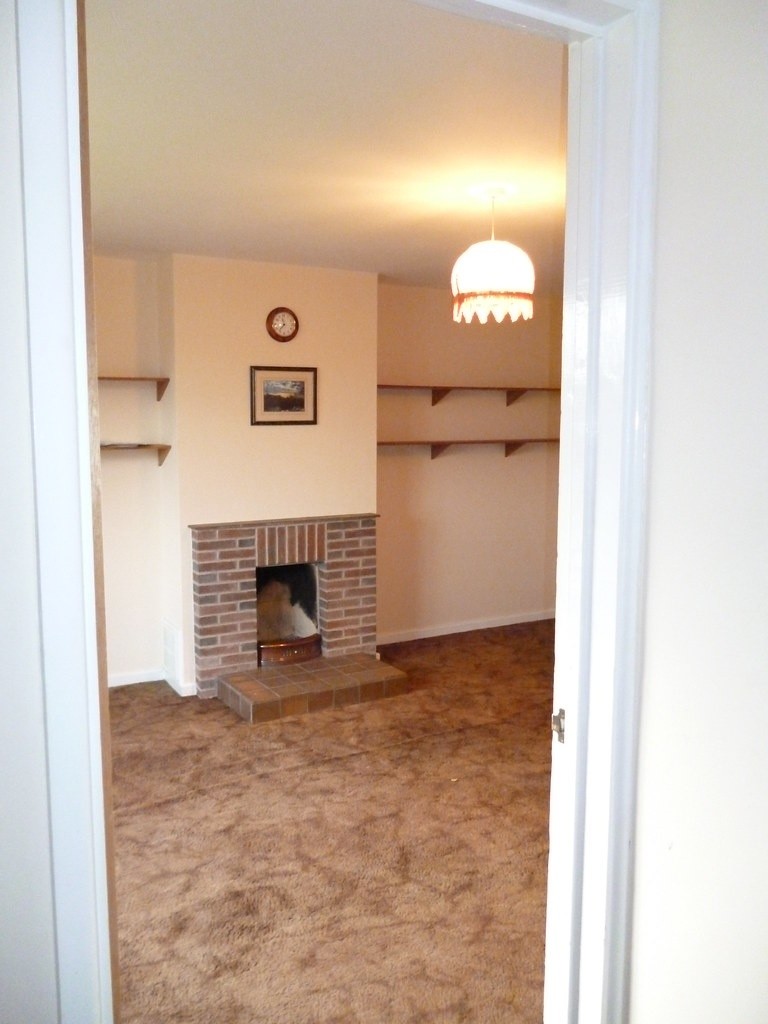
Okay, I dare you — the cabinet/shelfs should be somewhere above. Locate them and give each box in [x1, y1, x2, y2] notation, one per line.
[377, 384, 561, 459]
[98, 377, 172, 466]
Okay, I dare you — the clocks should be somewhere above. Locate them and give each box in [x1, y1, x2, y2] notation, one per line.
[266, 307, 299, 342]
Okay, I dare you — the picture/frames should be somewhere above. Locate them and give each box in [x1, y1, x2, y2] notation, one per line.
[251, 366, 317, 425]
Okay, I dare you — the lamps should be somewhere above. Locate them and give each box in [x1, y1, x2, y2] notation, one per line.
[450, 196, 536, 324]
